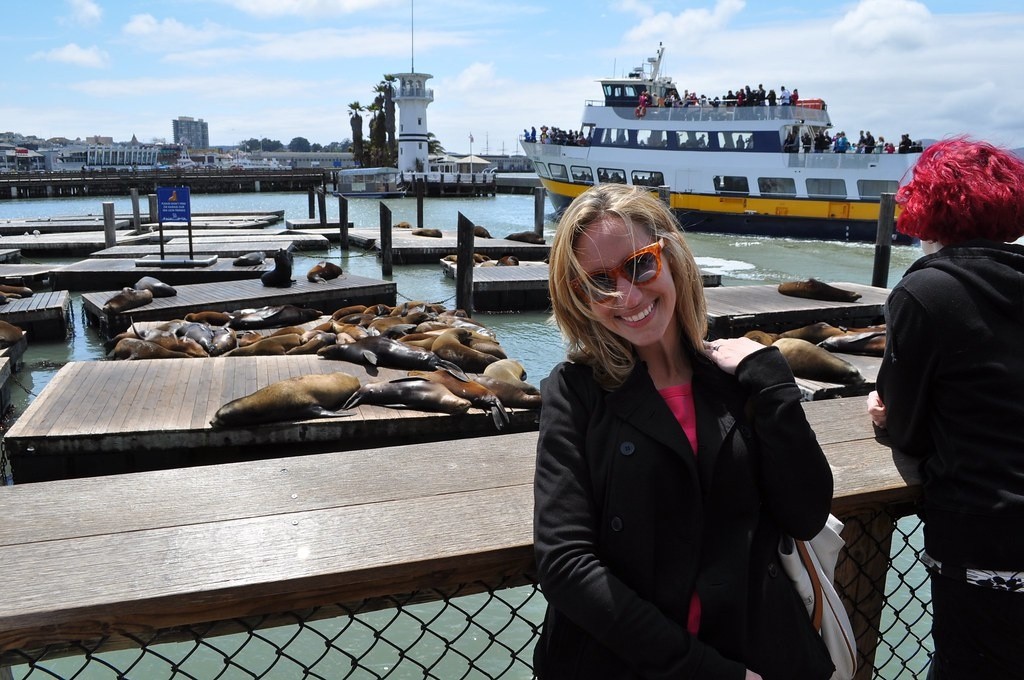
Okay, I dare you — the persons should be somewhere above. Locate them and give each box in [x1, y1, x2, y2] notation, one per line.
[864, 132, 1024, 680]
[713, 176, 721, 190]
[572, 169, 654, 187]
[522, 84, 924, 154]
[532, 181, 839, 679]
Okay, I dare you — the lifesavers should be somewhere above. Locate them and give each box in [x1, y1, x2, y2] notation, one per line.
[634, 104, 647, 118]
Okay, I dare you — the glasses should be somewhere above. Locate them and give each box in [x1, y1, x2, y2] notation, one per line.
[569, 238, 668, 306]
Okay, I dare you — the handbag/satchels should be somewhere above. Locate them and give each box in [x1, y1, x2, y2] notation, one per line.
[778, 509, 859, 680]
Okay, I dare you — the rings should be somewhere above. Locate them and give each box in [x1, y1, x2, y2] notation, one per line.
[714, 344, 722, 351]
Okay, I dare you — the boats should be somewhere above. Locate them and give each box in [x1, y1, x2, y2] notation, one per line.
[516, 41, 925, 246]
[331, 167, 408, 199]
